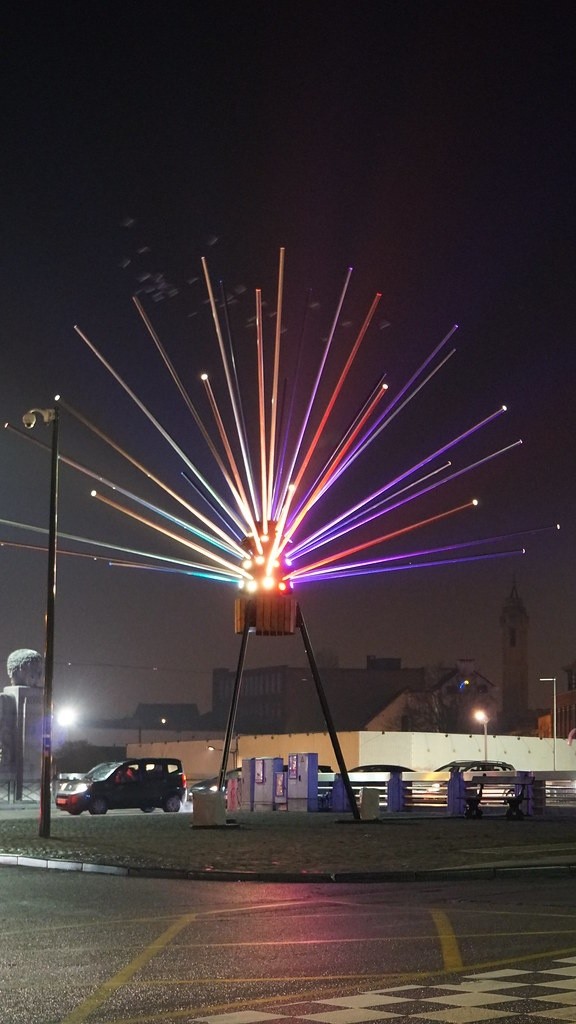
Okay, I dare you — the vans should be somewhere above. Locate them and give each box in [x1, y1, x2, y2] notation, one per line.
[55, 757, 186, 816]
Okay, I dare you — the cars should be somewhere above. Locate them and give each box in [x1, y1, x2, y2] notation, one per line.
[346, 764, 441, 801]
[190, 763, 334, 809]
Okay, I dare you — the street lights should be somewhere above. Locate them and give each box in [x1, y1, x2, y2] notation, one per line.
[474, 710, 489, 761]
[540, 678, 558, 772]
[20, 405, 60, 836]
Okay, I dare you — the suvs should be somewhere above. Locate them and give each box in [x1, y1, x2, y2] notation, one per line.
[433, 761, 515, 787]
